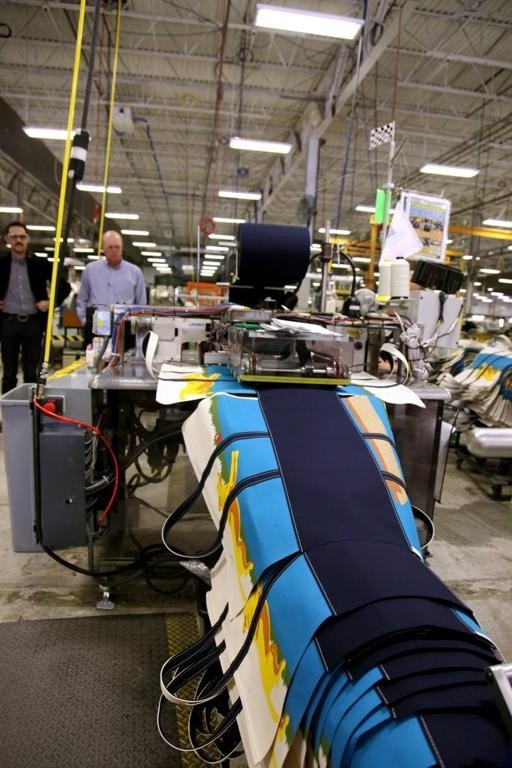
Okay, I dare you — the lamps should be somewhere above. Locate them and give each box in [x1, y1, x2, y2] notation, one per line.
[253, 3, 365, 41]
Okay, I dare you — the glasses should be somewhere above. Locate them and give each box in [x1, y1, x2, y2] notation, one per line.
[8, 235, 27, 240]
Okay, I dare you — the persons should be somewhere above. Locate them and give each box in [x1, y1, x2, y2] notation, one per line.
[75, 229, 149, 351]
[1, 222, 72, 394]
[378, 349, 401, 375]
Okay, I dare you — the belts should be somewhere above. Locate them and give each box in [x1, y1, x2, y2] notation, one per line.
[4, 314, 38, 322]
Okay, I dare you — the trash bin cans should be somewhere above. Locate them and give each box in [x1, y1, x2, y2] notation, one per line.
[0, 383, 49, 553]
